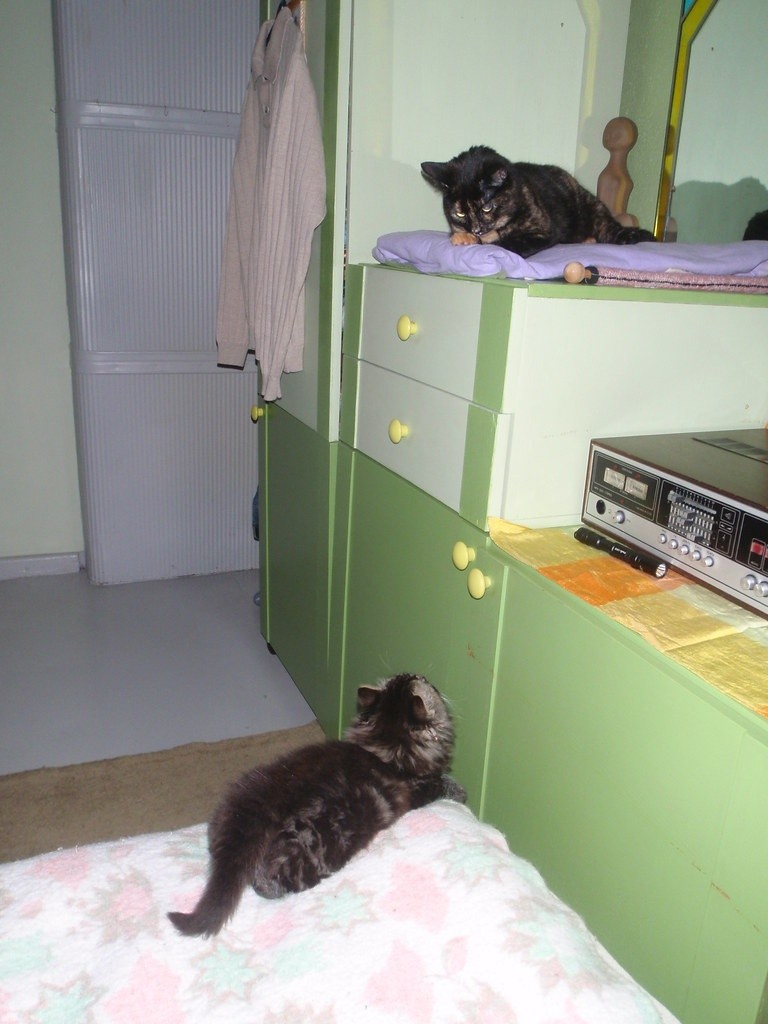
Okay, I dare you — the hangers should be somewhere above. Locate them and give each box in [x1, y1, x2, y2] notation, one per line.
[250, 0, 301, 74]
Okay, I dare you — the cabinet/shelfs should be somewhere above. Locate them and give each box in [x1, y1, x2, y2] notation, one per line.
[259, 0, 768, 1024]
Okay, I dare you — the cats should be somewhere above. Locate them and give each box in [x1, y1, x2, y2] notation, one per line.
[167, 672, 467, 939]
[421, 145, 658, 260]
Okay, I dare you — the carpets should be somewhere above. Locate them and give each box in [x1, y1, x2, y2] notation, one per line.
[0, 719, 327, 865]
